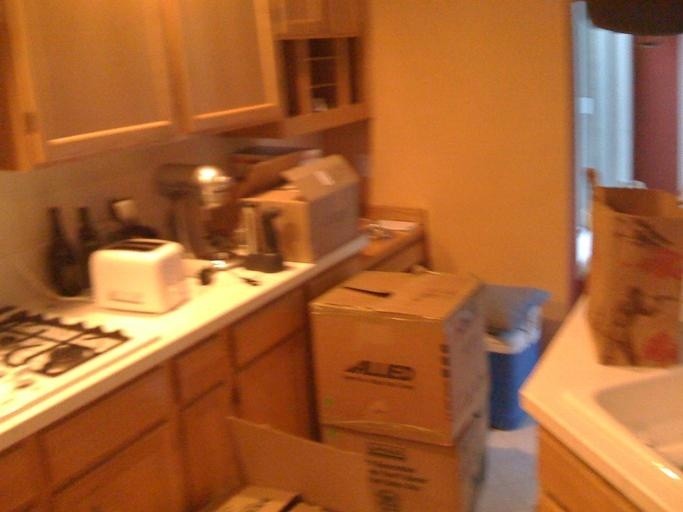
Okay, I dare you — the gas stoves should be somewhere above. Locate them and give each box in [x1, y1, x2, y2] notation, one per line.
[0, 304, 158, 428]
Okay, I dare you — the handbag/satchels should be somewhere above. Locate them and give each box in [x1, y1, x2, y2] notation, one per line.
[586, 168, 683, 366]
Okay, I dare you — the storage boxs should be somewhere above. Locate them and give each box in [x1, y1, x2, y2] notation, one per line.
[174, 149, 489, 512]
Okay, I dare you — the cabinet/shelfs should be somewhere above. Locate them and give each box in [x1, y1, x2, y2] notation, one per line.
[0, 364, 188, 512]
[0, 0, 283, 173]
[536, 427, 641, 512]
[270, 0, 369, 135]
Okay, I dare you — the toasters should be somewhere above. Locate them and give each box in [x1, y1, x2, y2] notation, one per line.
[85, 237, 187, 314]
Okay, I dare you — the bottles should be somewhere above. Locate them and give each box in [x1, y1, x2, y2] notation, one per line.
[111, 198, 160, 239]
[74, 206, 103, 253]
[41, 205, 83, 295]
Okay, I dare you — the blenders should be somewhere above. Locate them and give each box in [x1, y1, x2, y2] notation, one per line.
[152, 161, 244, 273]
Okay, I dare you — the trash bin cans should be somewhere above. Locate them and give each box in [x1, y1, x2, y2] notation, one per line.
[483, 282, 551, 432]
